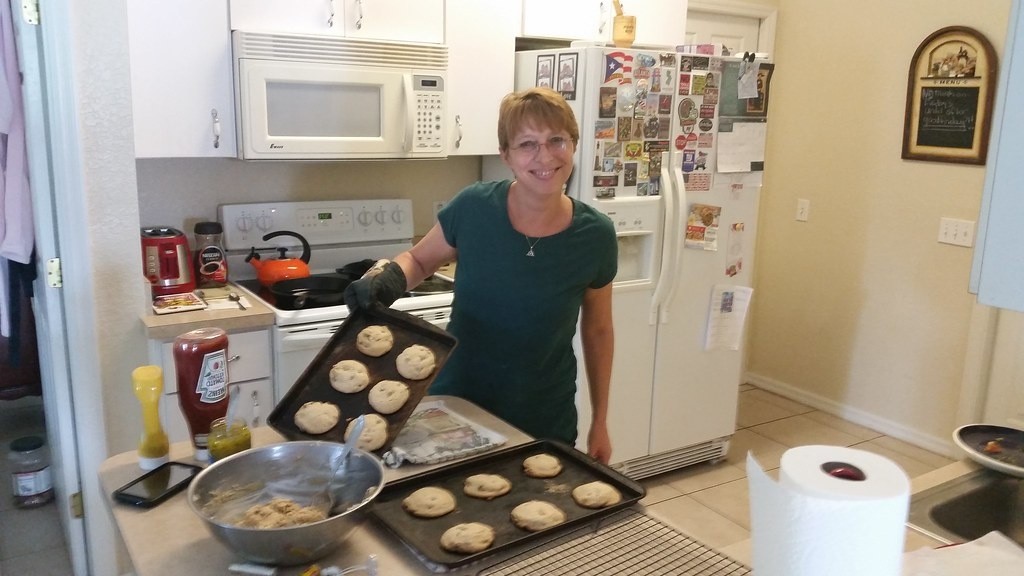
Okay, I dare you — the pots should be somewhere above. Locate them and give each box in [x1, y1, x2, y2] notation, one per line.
[269, 278, 353, 311]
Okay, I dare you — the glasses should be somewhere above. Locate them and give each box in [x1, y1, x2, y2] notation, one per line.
[503, 137, 577, 154]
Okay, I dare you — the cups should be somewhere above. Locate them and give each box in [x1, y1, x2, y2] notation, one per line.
[613, 15, 636, 47]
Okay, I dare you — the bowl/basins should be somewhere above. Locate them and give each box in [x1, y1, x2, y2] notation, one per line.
[186, 441, 385, 564]
[334, 259, 377, 279]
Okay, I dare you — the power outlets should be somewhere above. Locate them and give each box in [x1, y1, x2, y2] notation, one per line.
[937, 217, 976, 248]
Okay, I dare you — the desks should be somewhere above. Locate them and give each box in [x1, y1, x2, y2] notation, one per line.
[98, 396, 755, 576]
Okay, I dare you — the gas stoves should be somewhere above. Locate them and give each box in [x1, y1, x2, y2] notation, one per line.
[216, 198, 456, 326]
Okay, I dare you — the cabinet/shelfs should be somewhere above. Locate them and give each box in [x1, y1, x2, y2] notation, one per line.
[130, 0, 689, 159]
[139, 284, 272, 450]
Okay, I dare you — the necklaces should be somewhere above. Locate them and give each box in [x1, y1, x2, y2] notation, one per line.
[516, 213, 556, 257]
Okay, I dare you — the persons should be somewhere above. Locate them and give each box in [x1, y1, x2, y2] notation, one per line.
[341, 86, 619, 468]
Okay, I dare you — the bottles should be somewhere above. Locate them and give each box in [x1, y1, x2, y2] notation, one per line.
[132, 365, 170, 469]
[172, 327, 230, 460]
[193, 222, 227, 288]
[8, 437, 55, 508]
[209, 416, 249, 465]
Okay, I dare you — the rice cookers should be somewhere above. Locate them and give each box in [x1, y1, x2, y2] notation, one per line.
[140, 226, 196, 296]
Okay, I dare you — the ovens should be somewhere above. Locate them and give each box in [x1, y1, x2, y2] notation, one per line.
[272, 307, 453, 406]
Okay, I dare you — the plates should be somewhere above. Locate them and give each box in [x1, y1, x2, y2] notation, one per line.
[952, 424, 1024, 478]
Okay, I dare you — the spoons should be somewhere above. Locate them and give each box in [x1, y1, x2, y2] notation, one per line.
[229, 293, 246, 311]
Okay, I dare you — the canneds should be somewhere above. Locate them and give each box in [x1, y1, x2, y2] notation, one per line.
[207, 417, 252, 464]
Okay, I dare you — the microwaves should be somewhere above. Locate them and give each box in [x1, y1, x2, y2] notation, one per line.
[232, 28, 447, 161]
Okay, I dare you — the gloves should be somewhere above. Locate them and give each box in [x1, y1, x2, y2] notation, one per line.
[343, 259, 408, 314]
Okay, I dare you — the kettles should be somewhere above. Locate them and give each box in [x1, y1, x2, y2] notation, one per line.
[247, 231, 311, 287]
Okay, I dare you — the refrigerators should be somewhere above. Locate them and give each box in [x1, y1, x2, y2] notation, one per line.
[480, 49, 771, 480]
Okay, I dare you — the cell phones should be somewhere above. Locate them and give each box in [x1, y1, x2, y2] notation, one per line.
[113, 461, 203, 507]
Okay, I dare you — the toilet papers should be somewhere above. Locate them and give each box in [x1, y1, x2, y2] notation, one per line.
[743, 441, 913, 576]
